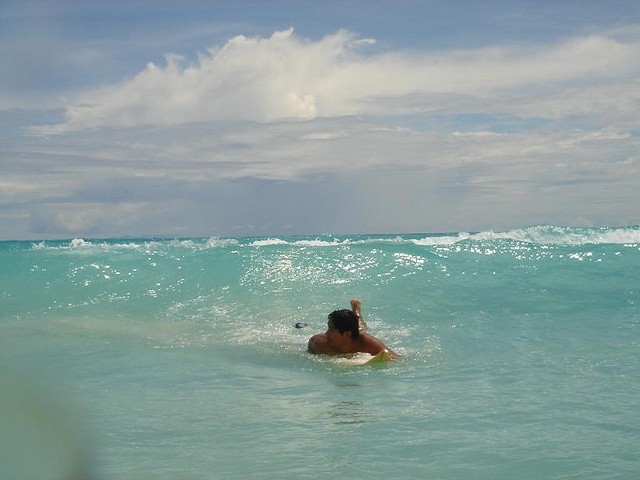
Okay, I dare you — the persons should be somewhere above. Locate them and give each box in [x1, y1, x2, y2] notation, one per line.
[309, 299, 398, 358]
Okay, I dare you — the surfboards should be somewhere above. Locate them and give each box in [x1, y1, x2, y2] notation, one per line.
[339, 351, 392, 366]
